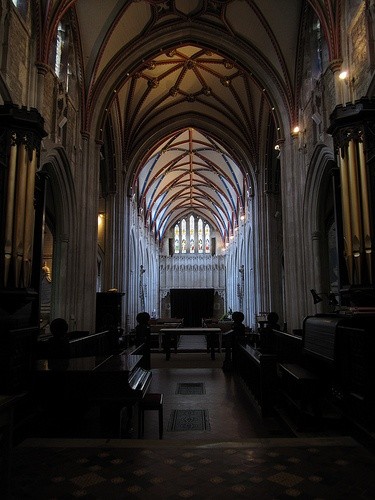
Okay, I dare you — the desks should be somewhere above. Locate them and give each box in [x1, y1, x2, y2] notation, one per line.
[160, 328, 221, 360]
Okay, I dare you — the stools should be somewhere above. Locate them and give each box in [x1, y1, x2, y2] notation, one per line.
[137, 392, 164, 440]
[273, 364, 317, 419]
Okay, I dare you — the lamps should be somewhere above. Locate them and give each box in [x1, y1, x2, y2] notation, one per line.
[311, 289, 339, 306]
